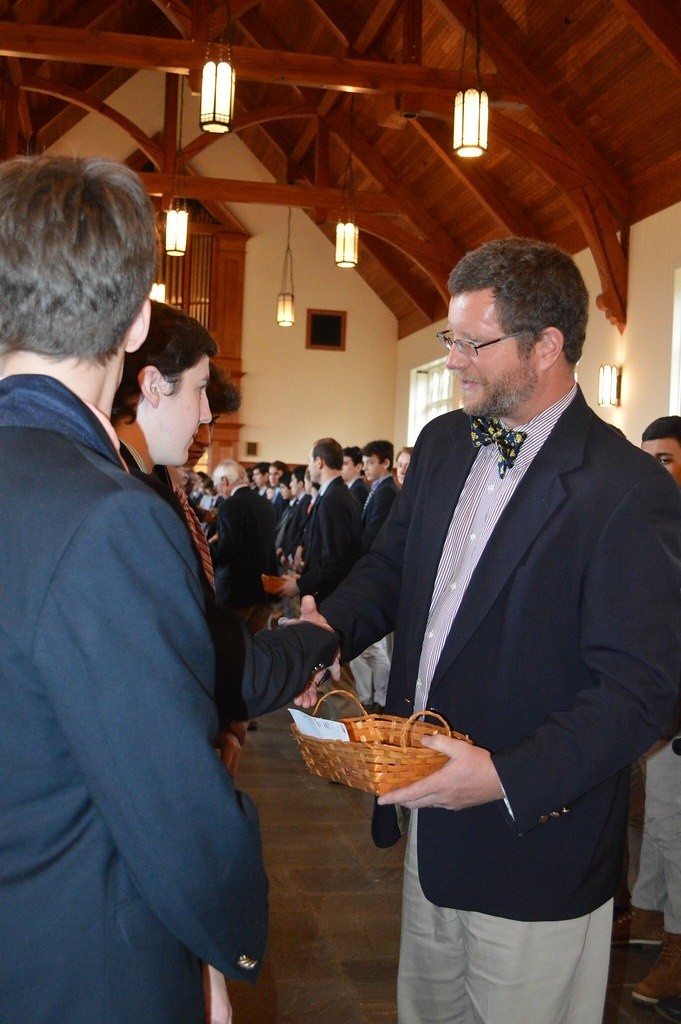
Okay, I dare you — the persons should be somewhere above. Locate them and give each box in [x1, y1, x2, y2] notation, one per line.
[0, 152, 339, 1024]
[184, 415, 681, 1003]
[273, 238, 680, 1023]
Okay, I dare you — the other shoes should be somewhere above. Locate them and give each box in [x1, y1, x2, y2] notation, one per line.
[654, 997, 681, 1023]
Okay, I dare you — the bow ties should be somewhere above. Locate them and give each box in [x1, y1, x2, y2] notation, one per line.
[469, 414, 526, 480]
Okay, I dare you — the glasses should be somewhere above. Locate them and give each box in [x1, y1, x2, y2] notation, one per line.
[436, 330, 535, 357]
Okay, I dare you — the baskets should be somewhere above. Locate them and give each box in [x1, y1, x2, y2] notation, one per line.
[290, 689, 473, 796]
[261, 573, 283, 592]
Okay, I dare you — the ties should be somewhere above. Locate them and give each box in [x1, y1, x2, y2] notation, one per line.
[175, 485, 215, 591]
[363, 489, 375, 511]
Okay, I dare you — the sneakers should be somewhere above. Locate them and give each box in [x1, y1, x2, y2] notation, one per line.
[611, 907, 664, 943]
[632, 930, 681, 1003]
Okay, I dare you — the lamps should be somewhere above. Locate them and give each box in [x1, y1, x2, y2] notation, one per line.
[276, 206, 295, 327]
[166, 75, 189, 257]
[454, 0, 488, 158]
[597, 364, 621, 408]
[198, 0, 237, 134]
[335, 93, 360, 270]
